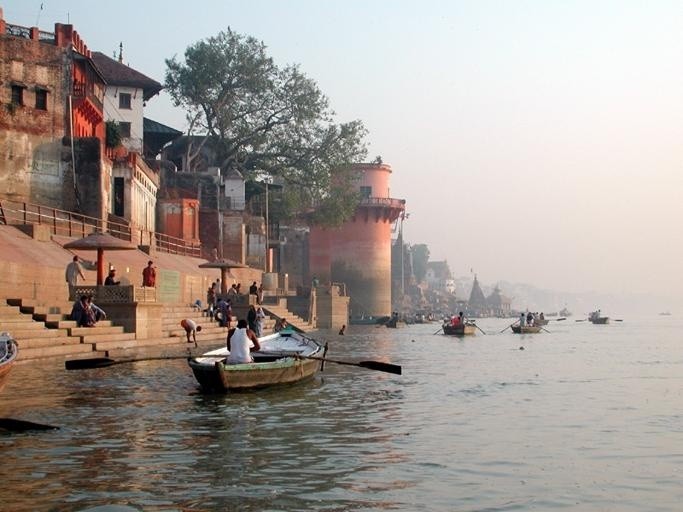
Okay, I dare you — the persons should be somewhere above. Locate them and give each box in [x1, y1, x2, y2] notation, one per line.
[142, 261, 156, 287]
[105, 270, 120, 285]
[207, 278, 265, 337]
[274, 318, 287, 331]
[69, 295, 106, 327]
[339, 325, 345, 335]
[520, 311, 544, 327]
[458, 312, 463, 318]
[227, 319, 261, 363]
[181, 319, 201, 347]
[65, 256, 85, 299]
[312, 277, 319, 284]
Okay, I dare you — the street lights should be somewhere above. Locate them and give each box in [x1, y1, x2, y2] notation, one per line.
[263, 173, 275, 250]
[399, 210, 410, 294]
[207, 166, 223, 257]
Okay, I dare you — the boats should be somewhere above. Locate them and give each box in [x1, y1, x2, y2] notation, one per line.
[184, 325, 327, 391]
[545, 312, 556, 316]
[348, 314, 379, 325]
[440, 324, 478, 336]
[588, 318, 591, 322]
[506, 323, 541, 334]
[404, 308, 518, 325]
[590, 316, 609, 324]
[534, 319, 549, 325]
[657, 311, 671, 315]
[0, 330, 20, 396]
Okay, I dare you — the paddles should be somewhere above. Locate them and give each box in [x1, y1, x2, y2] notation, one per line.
[64, 355, 229, 370]
[260, 352, 401, 375]
